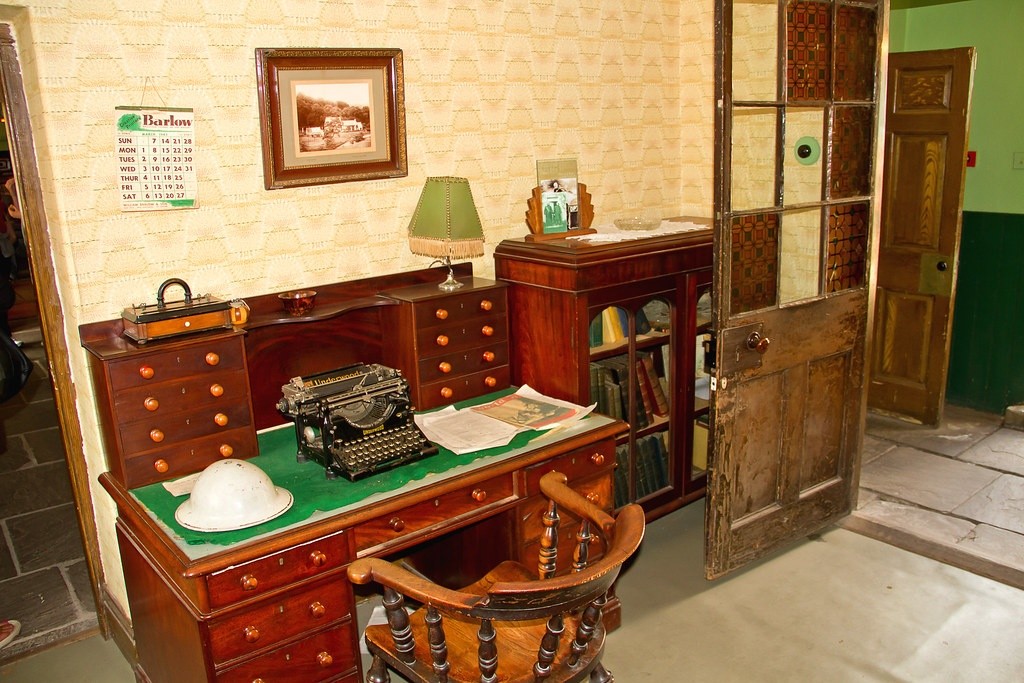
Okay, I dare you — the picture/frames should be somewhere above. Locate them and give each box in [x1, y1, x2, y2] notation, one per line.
[254, 47, 408, 191]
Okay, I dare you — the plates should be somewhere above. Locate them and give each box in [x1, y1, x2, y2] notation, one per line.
[615, 217, 663, 230]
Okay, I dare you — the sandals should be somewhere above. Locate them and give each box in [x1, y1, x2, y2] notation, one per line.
[0, 620, 21, 648]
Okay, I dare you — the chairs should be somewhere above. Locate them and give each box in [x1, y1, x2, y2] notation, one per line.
[345, 472, 645, 683]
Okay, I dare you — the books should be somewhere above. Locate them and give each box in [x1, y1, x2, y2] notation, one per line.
[588, 306, 670, 509]
[469, 393, 580, 432]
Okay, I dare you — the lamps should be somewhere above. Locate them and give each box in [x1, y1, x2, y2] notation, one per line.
[407, 176, 486, 290]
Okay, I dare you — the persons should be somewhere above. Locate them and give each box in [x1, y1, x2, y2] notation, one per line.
[0, 618, 22, 650]
[544, 180, 568, 228]
[0, 174, 24, 349]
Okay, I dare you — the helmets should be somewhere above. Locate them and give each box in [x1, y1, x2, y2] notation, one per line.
[174, 458, 293, 533]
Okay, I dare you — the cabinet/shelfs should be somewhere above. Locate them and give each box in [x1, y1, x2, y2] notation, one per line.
[117, 437, 617, 683]
[379, 287, 510, 411]
[89, 335, 258, 489]
[495, 235, 712, 522]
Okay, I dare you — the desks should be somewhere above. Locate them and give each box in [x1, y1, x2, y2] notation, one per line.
[96, 382, 630, 574]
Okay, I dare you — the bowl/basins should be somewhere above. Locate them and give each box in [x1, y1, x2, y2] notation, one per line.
[279, 291, 318, 317]
